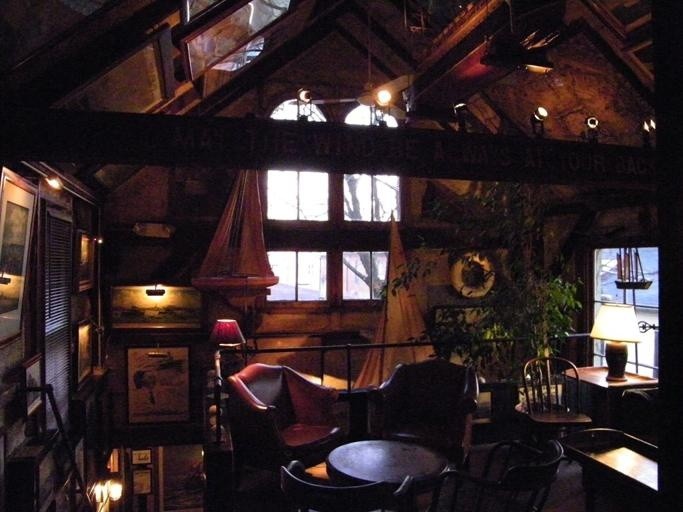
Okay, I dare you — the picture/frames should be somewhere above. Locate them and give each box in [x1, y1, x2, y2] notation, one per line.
[127, 348, 192, 421]
[81, 233, 91, 262]
[0, 166, 40, 347]
[131, 469, 153, 495]
[132, 449, 152, 464]
[77, 319, 92, 383]
[19, 353, 46, 419]
[110, 286, 200, 329]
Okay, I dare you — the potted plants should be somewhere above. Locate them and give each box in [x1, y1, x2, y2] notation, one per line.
[374, 176, 586, 415]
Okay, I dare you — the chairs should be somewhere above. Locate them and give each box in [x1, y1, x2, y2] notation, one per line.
[480, 436, 564, 512]
[338, 361, 478, 508]
[522, 356, 592, 463]
[281, 461, 414, 512]
[228, 363, 344, 485]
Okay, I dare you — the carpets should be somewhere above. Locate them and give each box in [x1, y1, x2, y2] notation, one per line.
[206, 421, 566, 512]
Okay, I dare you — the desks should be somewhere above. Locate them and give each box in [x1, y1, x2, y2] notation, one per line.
[564, 428, 659, 512]
[561, 367, 658, 429]
[325, 439, 449, 512]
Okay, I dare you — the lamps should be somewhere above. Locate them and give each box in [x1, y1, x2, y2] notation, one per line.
[373, 91, 391, 127]
[297, 87, 311, 121]
[531, 107, 548, 134]
[586, 117, 599, 141]
[454, 103, 465, 130]
[209, 319, 246, 443]
[589, 304, 642, 383]
